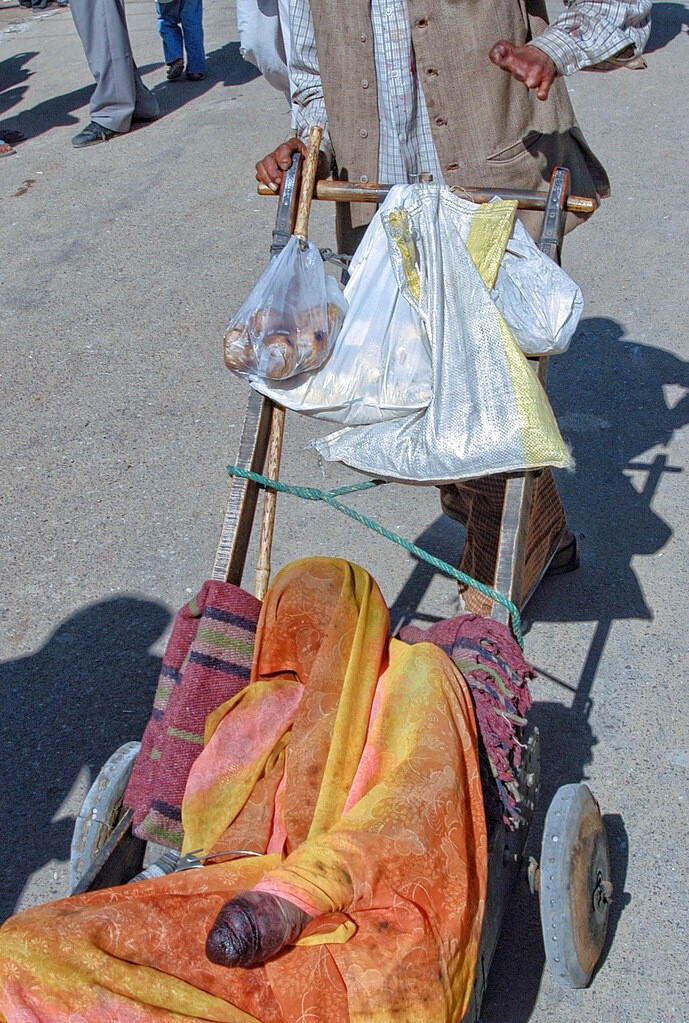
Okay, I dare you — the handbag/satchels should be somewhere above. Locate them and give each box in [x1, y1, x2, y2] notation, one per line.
[219, 234, 352, 383]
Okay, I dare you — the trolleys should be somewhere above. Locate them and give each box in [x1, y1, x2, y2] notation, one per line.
[48, 150, 619, 984]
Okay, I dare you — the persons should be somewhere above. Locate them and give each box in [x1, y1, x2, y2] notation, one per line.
[276, 0, 652, 634]
[0, 0, 205, 158]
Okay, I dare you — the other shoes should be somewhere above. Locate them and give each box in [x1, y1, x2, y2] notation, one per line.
[0, 129, 27, 158]
[166, 59, 184, 80]
[185, 72, 207, 81]
[71, 120, 121, 147]
[32, 7, 43, 13]
[131, 110, 159, 123]
